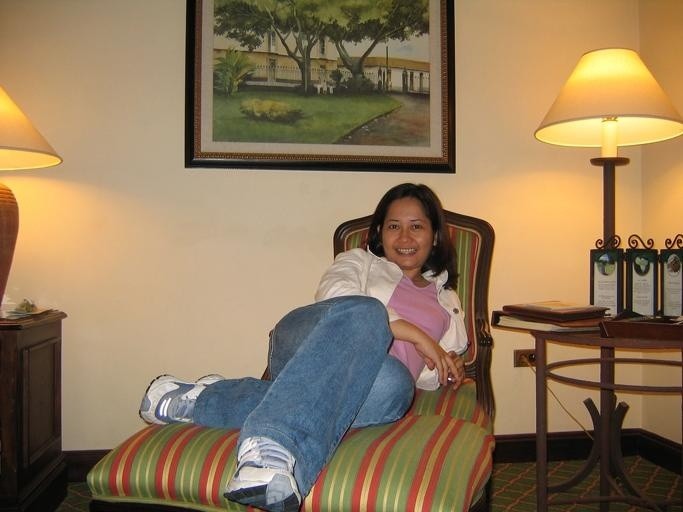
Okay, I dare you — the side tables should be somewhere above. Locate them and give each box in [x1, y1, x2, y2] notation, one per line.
[528, 330, 683, 511]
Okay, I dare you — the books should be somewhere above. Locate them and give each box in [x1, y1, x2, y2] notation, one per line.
[503, 299, 612, 322]
[489, 308, 602, 335]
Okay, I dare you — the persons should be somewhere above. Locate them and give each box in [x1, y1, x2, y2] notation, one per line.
[138, 182, 471, 511]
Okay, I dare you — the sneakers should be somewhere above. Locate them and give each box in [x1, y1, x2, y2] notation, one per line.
[223, 434, 302, 511]
[137, 373, 225, 426]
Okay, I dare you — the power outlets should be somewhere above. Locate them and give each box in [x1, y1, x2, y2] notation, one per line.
[514, 349, 537, 367]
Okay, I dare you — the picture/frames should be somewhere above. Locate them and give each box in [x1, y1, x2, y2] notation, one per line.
[185, 0, 455, 174]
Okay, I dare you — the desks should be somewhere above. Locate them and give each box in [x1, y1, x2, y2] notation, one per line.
[0, 311, 68, 512]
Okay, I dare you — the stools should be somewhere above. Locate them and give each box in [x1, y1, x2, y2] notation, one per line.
[86, 414, 496, 512]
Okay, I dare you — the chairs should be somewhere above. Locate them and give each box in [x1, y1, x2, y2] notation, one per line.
[261, 210, 496, 429]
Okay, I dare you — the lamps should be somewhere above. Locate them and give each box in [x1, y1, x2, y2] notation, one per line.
[0, 90, 62, 308]
[535, 49, 682, 511]
